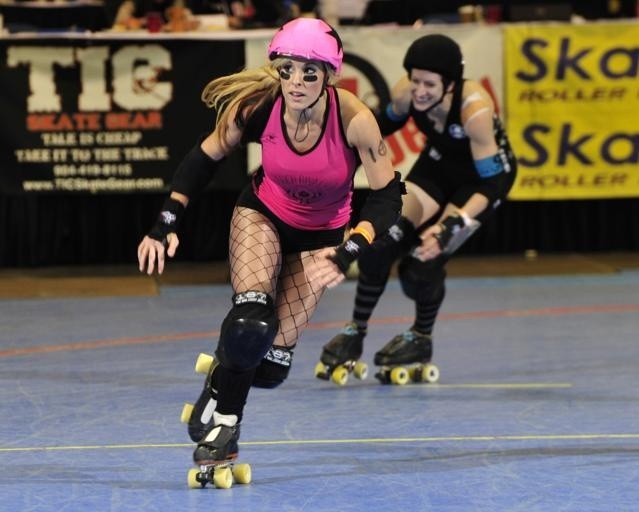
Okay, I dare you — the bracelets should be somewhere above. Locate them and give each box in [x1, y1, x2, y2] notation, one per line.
[352, 225, 374, 245]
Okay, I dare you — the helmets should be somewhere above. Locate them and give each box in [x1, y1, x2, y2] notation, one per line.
[268, 17, 343, 73]
[404, 34, 462, 78]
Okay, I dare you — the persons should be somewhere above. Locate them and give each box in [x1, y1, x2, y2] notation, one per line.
[319, 32, 519, 368]
[136, 14, 408, 467]
[1, 0, 639, 34]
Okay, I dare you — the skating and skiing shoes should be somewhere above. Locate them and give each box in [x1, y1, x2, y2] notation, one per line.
[315, 325, 369, 384]
[373, 330, 438, 382]
[179, 353, 221, 442]
[188, 412, 252, 490]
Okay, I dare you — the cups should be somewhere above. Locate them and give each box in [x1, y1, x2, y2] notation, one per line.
[487, 5, 499, 22]
[459, 5, 474, 22]
[148, 13, 161, 32]
